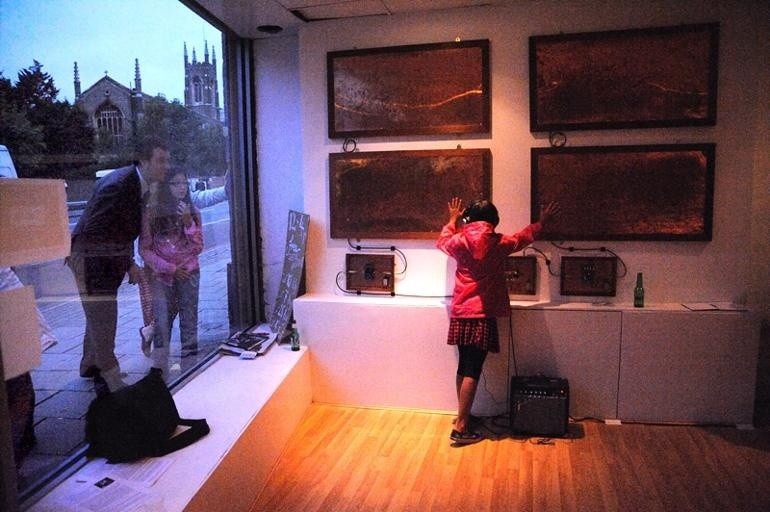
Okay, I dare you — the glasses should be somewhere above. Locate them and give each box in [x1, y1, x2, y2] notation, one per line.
[169, 181, 190, 188]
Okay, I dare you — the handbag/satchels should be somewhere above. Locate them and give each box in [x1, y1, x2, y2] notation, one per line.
[83, 367, 209, 463]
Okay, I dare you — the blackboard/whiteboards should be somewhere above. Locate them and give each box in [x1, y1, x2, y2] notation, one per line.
[269, 208, 310, 346]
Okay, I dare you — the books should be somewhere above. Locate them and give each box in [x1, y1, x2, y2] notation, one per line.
[218, 329, 279, 356]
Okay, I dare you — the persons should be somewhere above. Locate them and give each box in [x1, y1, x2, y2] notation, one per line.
[63, 139, 172, 396]
[434, 197, 564, 443]
[0, 266, 59, 466]
[195, 168, 225, 192]
[137, 167, 203, 380]
[137, 171, 232, 360]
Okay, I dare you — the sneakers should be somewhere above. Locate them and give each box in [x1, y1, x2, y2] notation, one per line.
[470, 415, 486, 427]
[450, 428, 483, 445]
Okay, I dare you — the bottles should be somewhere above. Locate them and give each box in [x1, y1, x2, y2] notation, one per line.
[633, 271, 644, 306]
[291, 320, 300, 350]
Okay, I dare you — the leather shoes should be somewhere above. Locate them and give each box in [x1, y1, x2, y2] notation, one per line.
[139, 327, 151, 357]
[174, 348, 197, 358]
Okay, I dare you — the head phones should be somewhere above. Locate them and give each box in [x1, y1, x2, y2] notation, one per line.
[466, 200, 499, 224]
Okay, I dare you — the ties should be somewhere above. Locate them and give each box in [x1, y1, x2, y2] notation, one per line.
[144, 188, 150, 207]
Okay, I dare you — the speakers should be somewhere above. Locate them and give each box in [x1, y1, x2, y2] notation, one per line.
[510, 376, 567, 438]
